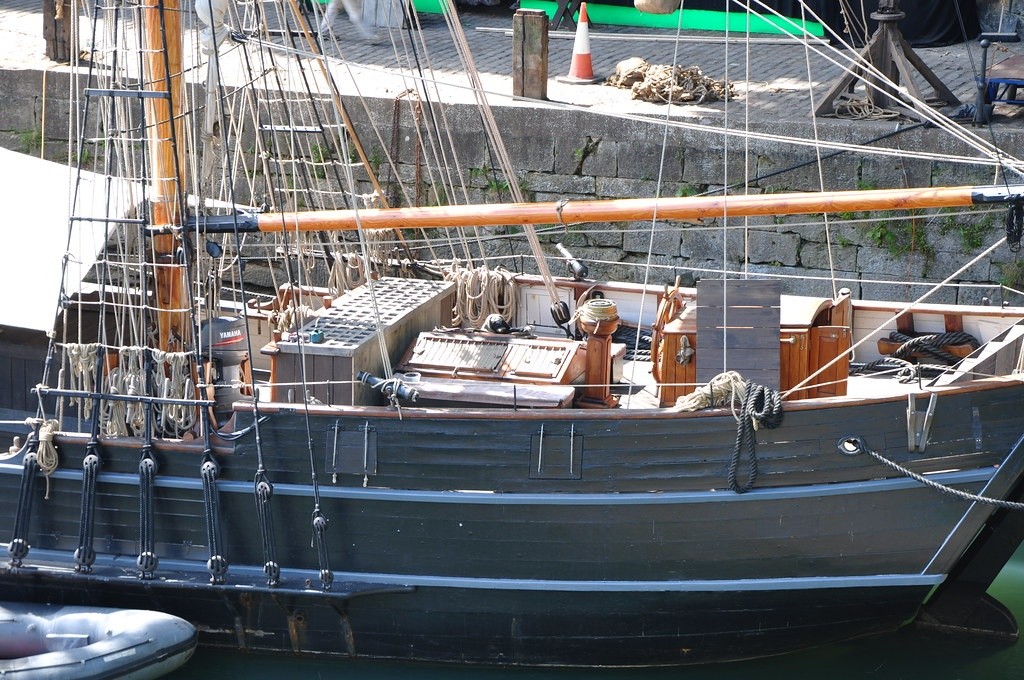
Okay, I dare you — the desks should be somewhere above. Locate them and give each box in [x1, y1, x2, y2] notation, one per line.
[260, 276, 457, 405]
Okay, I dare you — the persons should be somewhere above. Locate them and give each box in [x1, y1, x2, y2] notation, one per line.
[299, 0, 385, 44]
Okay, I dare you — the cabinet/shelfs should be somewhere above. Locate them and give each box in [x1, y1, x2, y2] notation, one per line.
[659, 294, 852, 408]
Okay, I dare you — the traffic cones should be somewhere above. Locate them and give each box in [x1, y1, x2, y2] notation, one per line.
[553, 0, 606, 87]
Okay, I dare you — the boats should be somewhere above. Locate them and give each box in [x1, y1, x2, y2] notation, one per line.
[0, 596, 198, 679]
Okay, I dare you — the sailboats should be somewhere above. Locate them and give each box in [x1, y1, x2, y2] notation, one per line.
[0, 0, 1024, 671]
